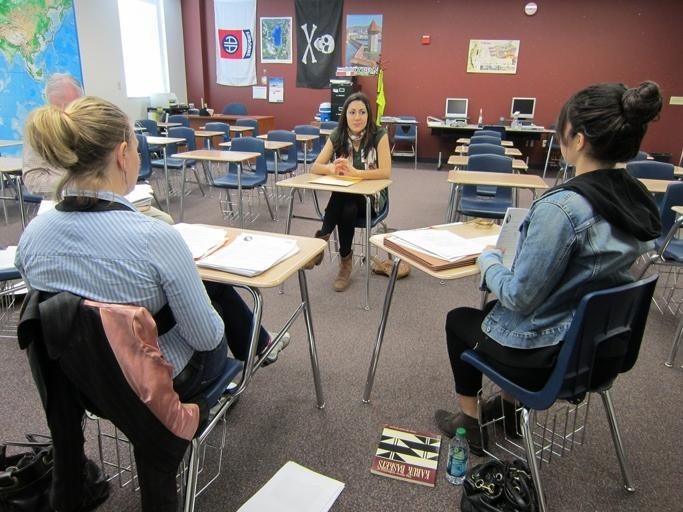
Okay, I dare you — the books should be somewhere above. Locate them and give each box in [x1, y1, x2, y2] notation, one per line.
[371, 423, 442, 487]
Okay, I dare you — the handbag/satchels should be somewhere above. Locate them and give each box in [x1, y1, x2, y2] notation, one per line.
[362, 254, 411, 280]
[0, 432, 111, 512]
[458, 458, 543, 512]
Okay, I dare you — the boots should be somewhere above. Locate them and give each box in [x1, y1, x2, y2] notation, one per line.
[301, 231, 331, 271]
[333, 251, 354, 293]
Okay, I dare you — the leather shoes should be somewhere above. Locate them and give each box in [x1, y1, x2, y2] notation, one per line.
[477, 392, 525, 441]
[433, 408, 489, 458]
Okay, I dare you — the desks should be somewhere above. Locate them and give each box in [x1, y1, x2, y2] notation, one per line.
[270, 169, 396, 314]
[360, 217, 513, 413]
[154, 220, 333, 413]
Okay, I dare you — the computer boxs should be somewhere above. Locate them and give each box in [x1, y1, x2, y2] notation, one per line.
[446, 118, 468, 126]
[511, 120, 532, 128]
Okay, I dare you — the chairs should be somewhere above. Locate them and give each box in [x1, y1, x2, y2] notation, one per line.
[426, 104, 682, 372]
[0, 102, 422, 233]
[11, 277, 265, 512]
[452, 270, 663, 510]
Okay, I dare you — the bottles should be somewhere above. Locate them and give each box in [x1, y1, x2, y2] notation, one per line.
[168, 92, 177, 108]
[444, 427, 470, 484]
[476, 108, 483, 128]
[497, 112, 503, 127]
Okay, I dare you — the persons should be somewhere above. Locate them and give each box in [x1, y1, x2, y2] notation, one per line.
[435, 81, 663, 455]
[303, 92, 391, 292]
[21, 73, 176, 225]
[15, 94, 291, 402]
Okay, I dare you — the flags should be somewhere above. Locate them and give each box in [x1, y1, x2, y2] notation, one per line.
[214, 0, 258, 86]
[294, 0, 343, 89]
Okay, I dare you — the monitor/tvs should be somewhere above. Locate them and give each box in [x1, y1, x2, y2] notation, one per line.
[510, 97, 536, 118]
[445, 98, 469, 119]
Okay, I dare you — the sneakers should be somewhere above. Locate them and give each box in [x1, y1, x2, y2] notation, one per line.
[259, 330, 291, 368]
